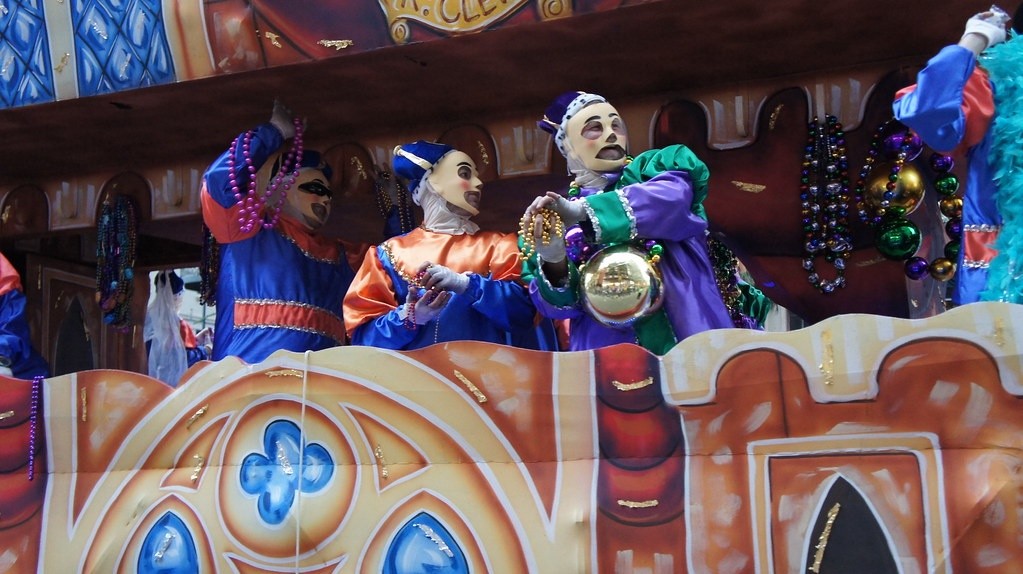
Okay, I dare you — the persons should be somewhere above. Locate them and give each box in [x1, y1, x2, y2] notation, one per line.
[515, 86, 734, 346]
[198, 84, 376, 362]
[0, 254, 50, 380]
[894, 9, 1023, 305]
[144, 267, 216, 388]
[344, 141, 563, 357]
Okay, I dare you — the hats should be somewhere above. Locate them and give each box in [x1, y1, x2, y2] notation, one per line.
[390, 138, 456, 206]
[538, 90, 608, 158]
[266, 146, 332, 181]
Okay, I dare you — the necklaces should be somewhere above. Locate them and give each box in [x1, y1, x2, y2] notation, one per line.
[563, 155, 666, 327]
[403, 258, 450, 343]
[29, 375, 44, 481]
[518, 201, 563, 262]
[227, 111, 304, 233]
[376, 171, 414, 234]
[94, 192, 139, 338]
[799, 111, 963, 296]
[199, 222, 220, 307]
